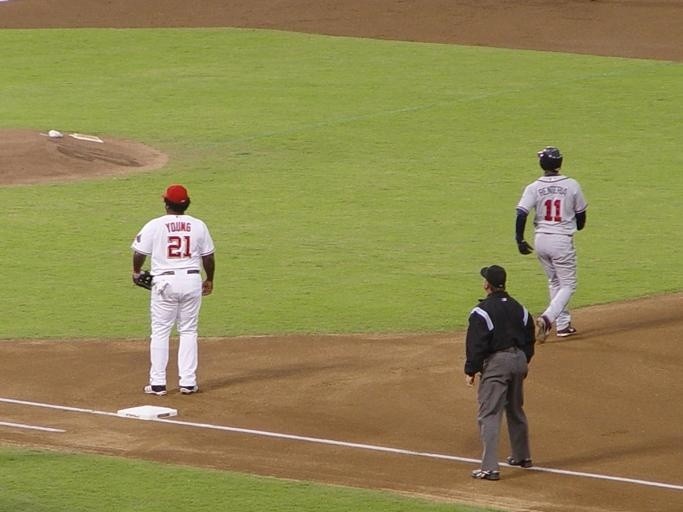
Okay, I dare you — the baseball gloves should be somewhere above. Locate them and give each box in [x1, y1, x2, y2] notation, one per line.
[133, 269, 153, 290]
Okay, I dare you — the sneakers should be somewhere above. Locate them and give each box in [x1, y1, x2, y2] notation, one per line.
[534, 316, 547, 344]
[142, 385, 168, 395]
[556, 325, 578, 337]
[179, 386, 199, 394]
[507, 455, 534, 469]
[471, 467, 501, 482]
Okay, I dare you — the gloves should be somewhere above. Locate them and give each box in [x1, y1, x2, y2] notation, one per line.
[517, 240, 534, 255]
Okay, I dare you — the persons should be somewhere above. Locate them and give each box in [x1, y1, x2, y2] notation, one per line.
[514, 144, 588, 343]
[129, 184, 215, 396]
[463, 263, 537, 481]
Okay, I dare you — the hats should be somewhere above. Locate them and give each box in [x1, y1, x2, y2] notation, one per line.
[162, 184, 189, 206]
[479, 265, 508, 289]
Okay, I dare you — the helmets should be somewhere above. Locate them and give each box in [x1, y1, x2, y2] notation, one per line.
[536, 145, 563, 167]
